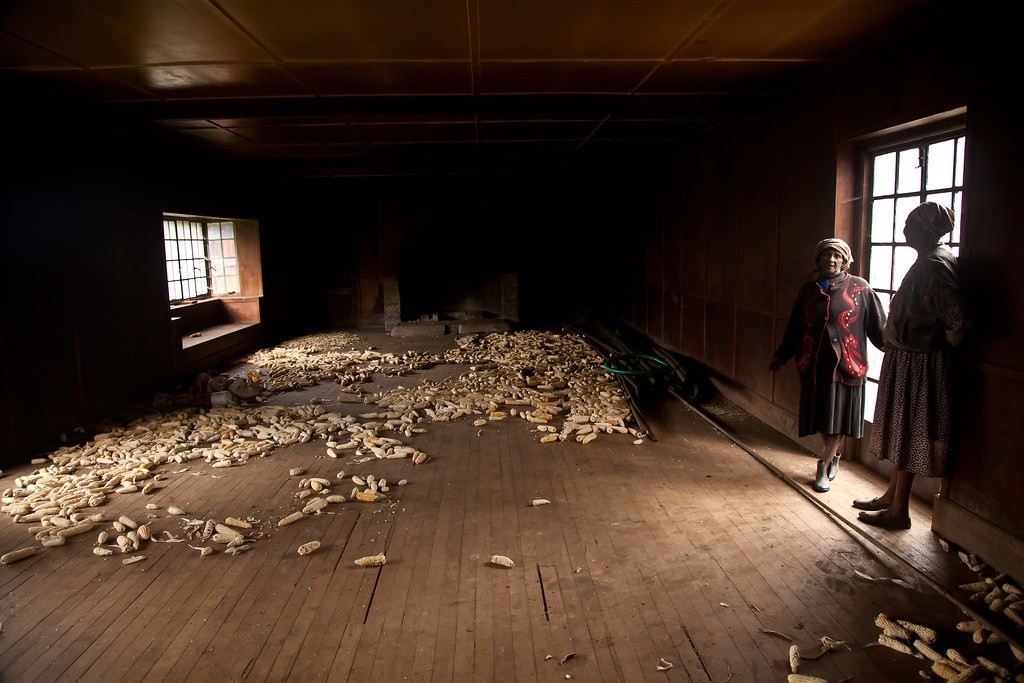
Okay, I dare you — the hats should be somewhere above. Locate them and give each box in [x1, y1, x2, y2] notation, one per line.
[811, 238, 855, 271]
[910, 202, 954, 239]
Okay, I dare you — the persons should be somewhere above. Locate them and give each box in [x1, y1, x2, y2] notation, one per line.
[767, 237, 888, 493]
[853, 200, 969, 532]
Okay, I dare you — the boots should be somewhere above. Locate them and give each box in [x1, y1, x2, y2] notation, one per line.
[814, 459, 830, 492]
[828, 453, 841, 481]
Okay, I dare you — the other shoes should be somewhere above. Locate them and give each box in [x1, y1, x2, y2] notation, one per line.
[853, 497, 911, 529]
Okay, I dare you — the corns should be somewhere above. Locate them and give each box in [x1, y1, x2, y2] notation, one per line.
[788, 538, 1024, 683]
[0, 330, 647, 566]
